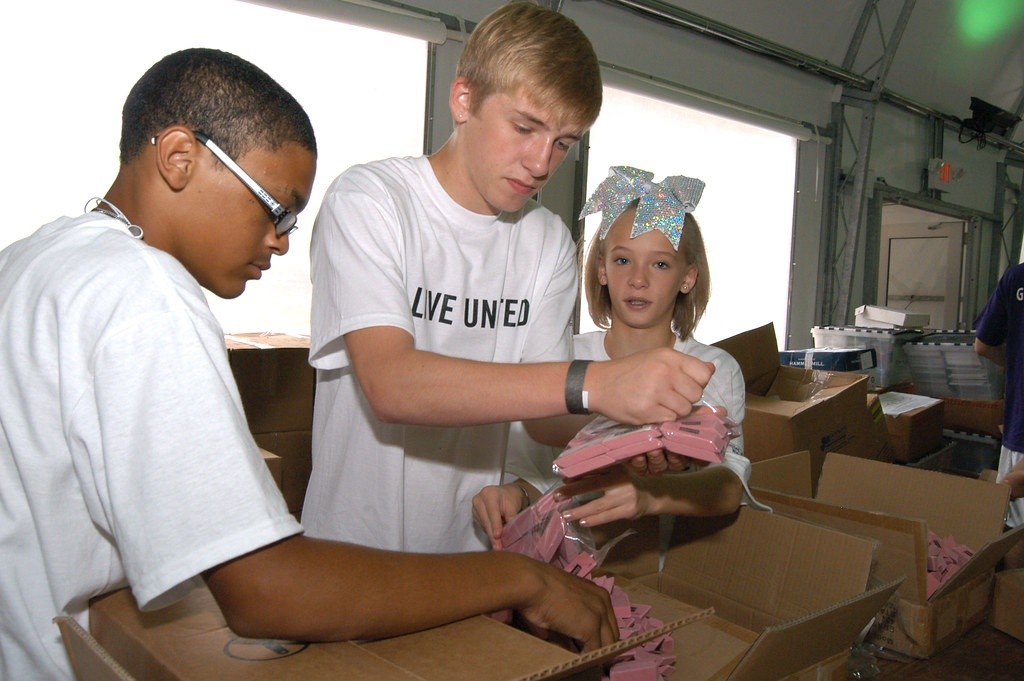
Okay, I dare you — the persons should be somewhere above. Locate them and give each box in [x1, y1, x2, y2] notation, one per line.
[973, 263, 1024, 534]
[472, 166, 772, 550]
[0, 47, 621, 681]
[302, 0, 715, 548]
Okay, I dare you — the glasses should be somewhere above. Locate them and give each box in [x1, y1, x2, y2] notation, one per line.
[151, 131, 299, 240]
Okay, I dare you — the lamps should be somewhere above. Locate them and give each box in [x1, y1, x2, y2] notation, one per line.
[961, 95, 1024, 137]
[928, 157, 966, 194]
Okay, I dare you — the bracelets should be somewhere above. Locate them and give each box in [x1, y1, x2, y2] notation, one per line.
[517, 483, 532, 508]
[565, 360, 593, 416]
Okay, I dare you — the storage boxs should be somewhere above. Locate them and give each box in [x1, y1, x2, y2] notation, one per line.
[52, 304, 1024, 681]
